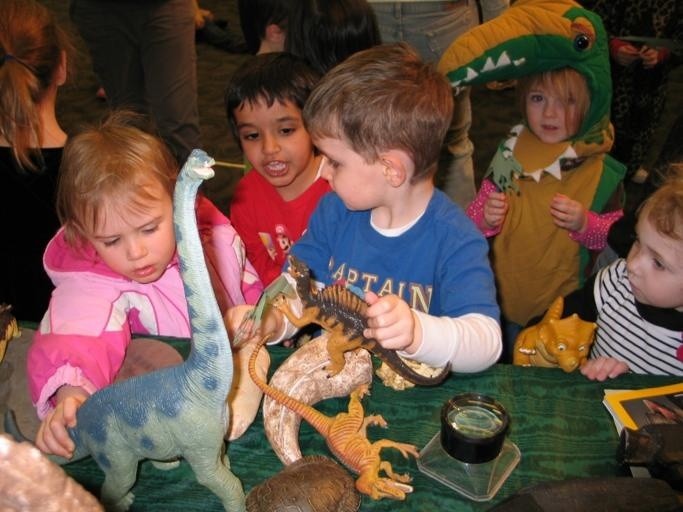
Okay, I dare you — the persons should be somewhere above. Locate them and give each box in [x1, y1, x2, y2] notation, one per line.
[27, 111, 266, 460]
[524, 163, 682, 382]
[467, 70, 623, 358]
[222, 42, 503, 375]
[257, 2, 682, 206]
[225, 53, 333, 288]
[0, 1, 67, 324]
[69, 1, 214, 169]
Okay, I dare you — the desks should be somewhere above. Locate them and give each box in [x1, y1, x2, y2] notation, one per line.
[18, 322, 683, 512]
